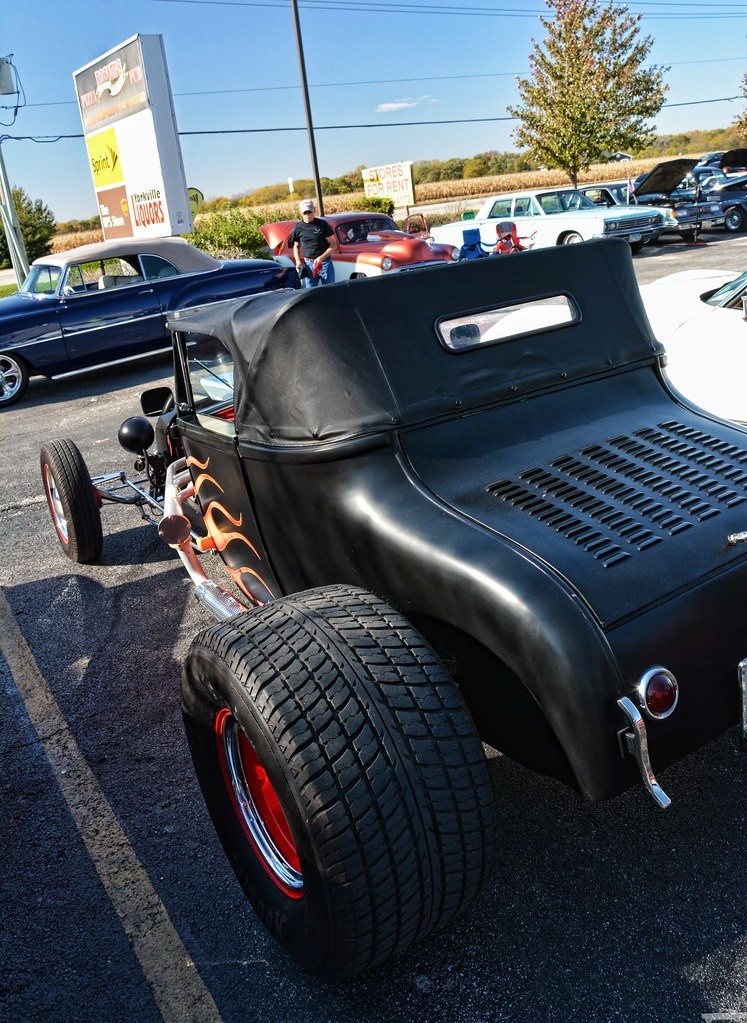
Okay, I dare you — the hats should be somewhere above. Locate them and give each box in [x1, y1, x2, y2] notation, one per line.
[299, 200, 314, 213]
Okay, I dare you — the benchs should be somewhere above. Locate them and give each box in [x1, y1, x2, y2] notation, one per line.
[97, 275, 116, 289]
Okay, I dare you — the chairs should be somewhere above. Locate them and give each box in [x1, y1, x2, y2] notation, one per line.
[159, 266, 178, 278]
[115, 275, 143, 287]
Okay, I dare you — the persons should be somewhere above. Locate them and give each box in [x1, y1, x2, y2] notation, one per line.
[292, 199, 341, 290]
[349, 223, 365, 243]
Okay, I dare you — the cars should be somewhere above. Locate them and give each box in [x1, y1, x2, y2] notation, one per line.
[699, 150, 747, 176]
[429, 188, 664, 253]
[259, 211, 462, 286]
[580, 158, 726, 245]
[42, 232, 744, 978]
[0, 238, 304, 408]
[631, 167, 747, 227]
[476, 269, 747, 424]
